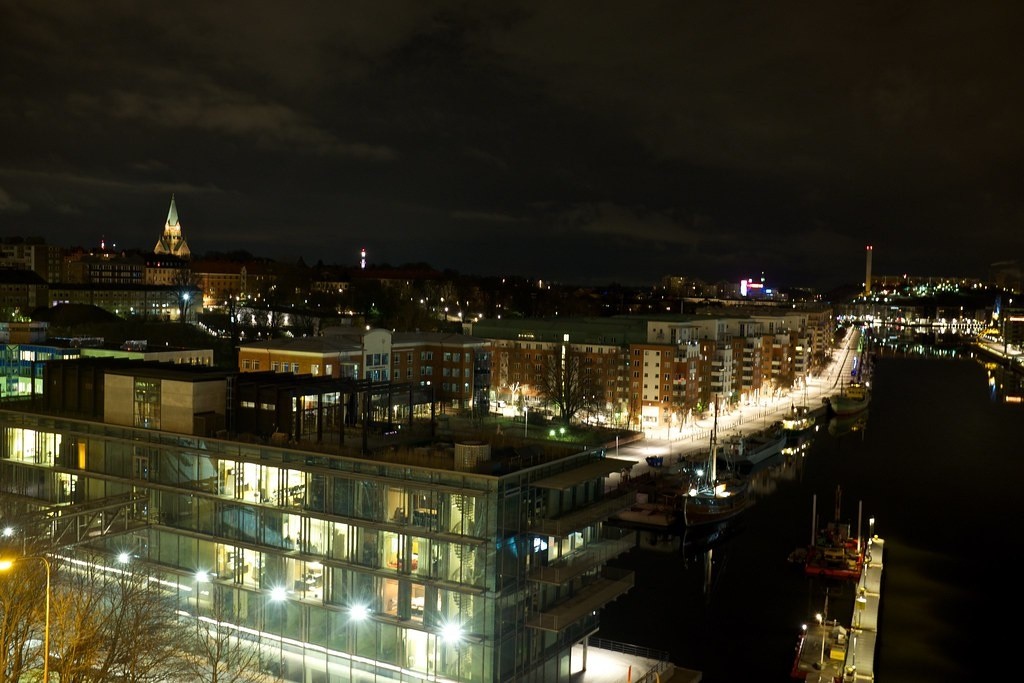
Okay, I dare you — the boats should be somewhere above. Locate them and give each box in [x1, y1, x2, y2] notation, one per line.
[781, 393, 816, 434]
[805, 484, 866, 585]
[716, 422, 787, 468]
[787, 547, 807, 564]
[676, 394, 749, 528]
[791, 586, 849, 683]
[828, 327, 874, 416]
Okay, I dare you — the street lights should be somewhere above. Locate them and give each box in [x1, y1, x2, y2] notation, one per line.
[615, 412, 620, 458]
[0, 557, 49, 683]
[523, 407, 528, 438]
